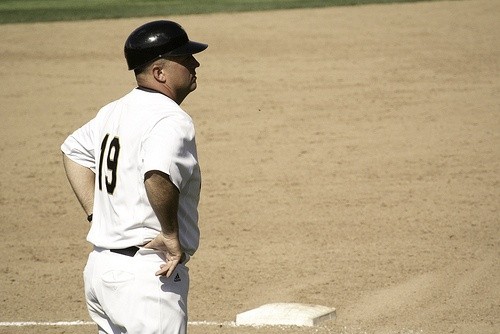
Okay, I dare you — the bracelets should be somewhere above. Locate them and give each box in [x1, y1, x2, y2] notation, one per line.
[86, 213, 93, 223]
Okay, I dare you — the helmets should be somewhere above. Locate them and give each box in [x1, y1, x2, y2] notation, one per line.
[124, 20, 208, 70]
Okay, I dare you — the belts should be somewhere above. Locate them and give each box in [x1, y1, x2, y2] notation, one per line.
[110, 246, 139, 257]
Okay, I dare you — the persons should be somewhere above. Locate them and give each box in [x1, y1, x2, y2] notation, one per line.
[61, 19, 208, 333]
